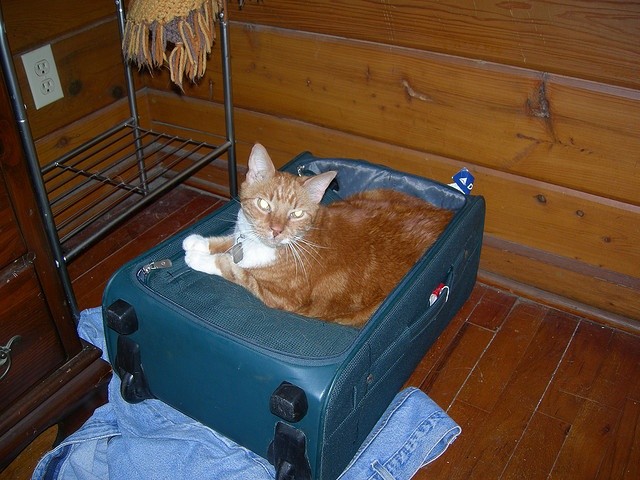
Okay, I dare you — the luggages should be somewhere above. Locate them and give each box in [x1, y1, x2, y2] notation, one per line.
[101, 149, 487, 480]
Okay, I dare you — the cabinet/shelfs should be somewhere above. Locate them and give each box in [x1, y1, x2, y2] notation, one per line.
[0, 0, 238, 320]
[1, 62, 113, 478]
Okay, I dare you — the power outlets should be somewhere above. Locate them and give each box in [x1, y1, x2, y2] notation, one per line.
[21, 43, 64, 110]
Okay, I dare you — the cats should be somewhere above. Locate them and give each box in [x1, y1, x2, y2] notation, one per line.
[182, 141, 457, 330]
